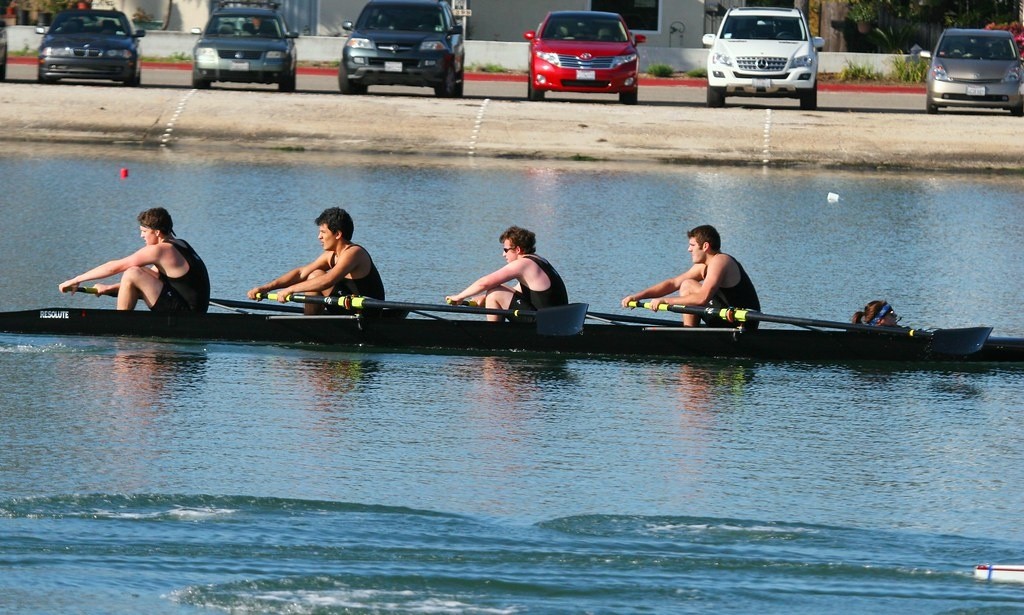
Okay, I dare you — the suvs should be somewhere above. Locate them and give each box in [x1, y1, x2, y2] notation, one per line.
[702, 6, 825, 110]
[336, 0, 465, 99]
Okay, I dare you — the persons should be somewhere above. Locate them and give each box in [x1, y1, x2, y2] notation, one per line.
[247, 207, 385, 315]
[622, 224, 760, 328]
[59, 207, 210, 313]
[445, 226, 568, 321]
[851, 300, 910, 335]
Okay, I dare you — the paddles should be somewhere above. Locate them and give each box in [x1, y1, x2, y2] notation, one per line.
[249, 291, 591, 337]
[626, 299, 995, 356]
[67, 285, 305, 314]
[446, 298, 685, 327]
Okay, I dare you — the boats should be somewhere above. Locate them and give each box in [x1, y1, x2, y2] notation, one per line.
[0, 303, 1023, 368]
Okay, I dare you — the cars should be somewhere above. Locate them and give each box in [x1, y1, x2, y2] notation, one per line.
[35, 8, 146, 85]
[919, 28, 1024, 116]
[190, 3, 300, 92]
[523, 10, 648, 105]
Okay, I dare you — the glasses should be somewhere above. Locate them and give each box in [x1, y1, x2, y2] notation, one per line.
[503, 247, 518, 253]
